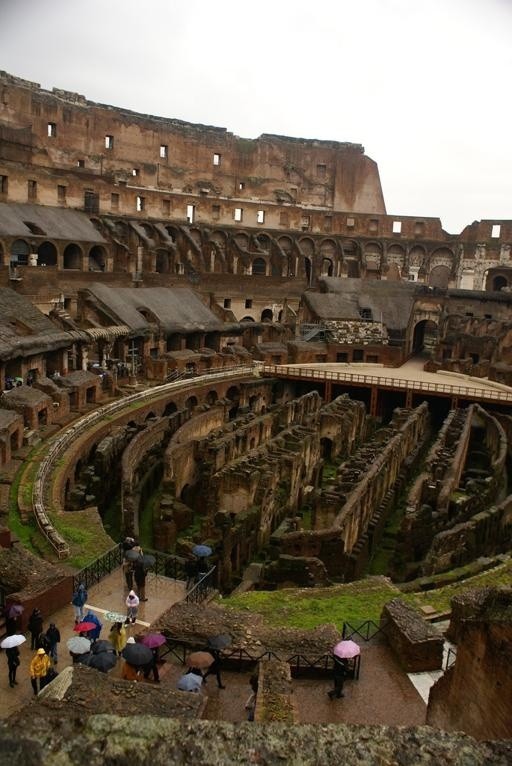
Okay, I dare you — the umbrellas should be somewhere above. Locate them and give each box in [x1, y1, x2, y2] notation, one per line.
[333, 639, 360, 659]
[191, 544, 213, 557]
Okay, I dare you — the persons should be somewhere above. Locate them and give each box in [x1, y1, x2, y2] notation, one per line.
[2, 594, 230, 706]
[184, 559, 198, 590]
[245, 662, 260, 707]
[124, 590, 142, 626]
[120, 535, 149, 603]
[72, 588, 88, 623]
[195, 556, 211, 585]
[332, 654, 351, 700]
[74, 579, 85, 608]
